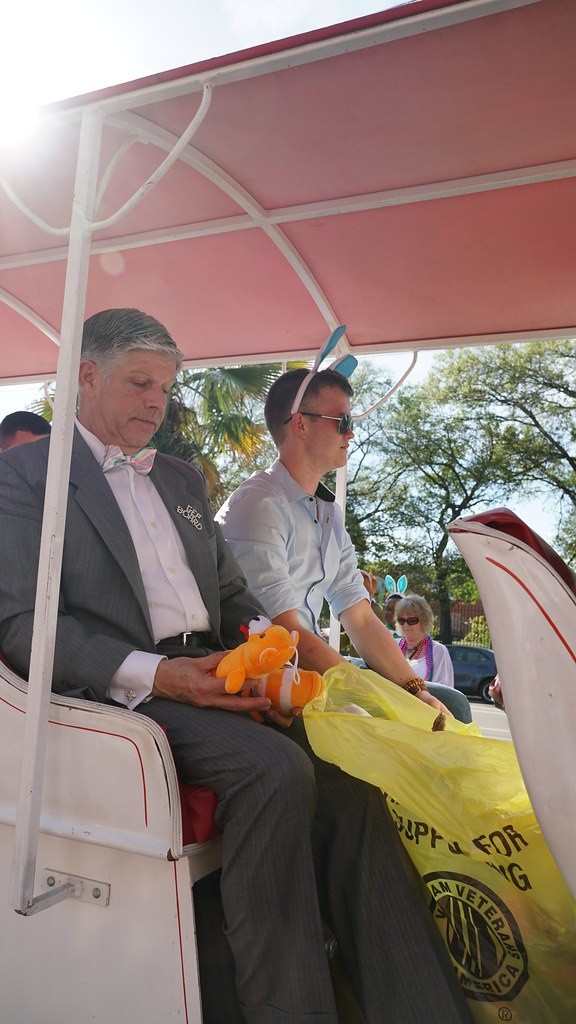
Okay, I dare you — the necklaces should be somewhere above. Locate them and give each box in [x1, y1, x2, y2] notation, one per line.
[399, 634, 434, 683]
[215, 368, 475, 725]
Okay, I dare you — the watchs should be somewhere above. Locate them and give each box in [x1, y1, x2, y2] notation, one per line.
[407, 685, 429, 695]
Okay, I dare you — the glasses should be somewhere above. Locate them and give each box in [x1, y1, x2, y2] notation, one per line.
[284, 411, 354, 435]
[396, 617, 419, 626]
[382, 605, 395, 613]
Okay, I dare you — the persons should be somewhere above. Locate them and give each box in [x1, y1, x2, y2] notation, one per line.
[0, 306, 478, 1024]
[383, 592, 405, 644]
[321, 599, 384, 658]
[395, 595, 455, 689]
[0, 412, 53, 451]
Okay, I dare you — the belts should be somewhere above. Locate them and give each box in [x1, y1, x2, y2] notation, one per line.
[157, 631, 215, 649]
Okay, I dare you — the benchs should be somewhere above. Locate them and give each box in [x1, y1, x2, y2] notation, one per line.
[0, 656, 223, 891]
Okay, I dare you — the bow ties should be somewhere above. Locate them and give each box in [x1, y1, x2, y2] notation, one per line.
[97, 442, 157, 476]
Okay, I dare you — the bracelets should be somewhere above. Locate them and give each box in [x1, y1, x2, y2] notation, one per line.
[402, 677, 426, 691]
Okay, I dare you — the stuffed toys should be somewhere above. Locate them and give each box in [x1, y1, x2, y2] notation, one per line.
[211, 615, 324, 718]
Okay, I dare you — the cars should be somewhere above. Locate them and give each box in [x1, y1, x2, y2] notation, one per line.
[441, 640, 501, 705]
